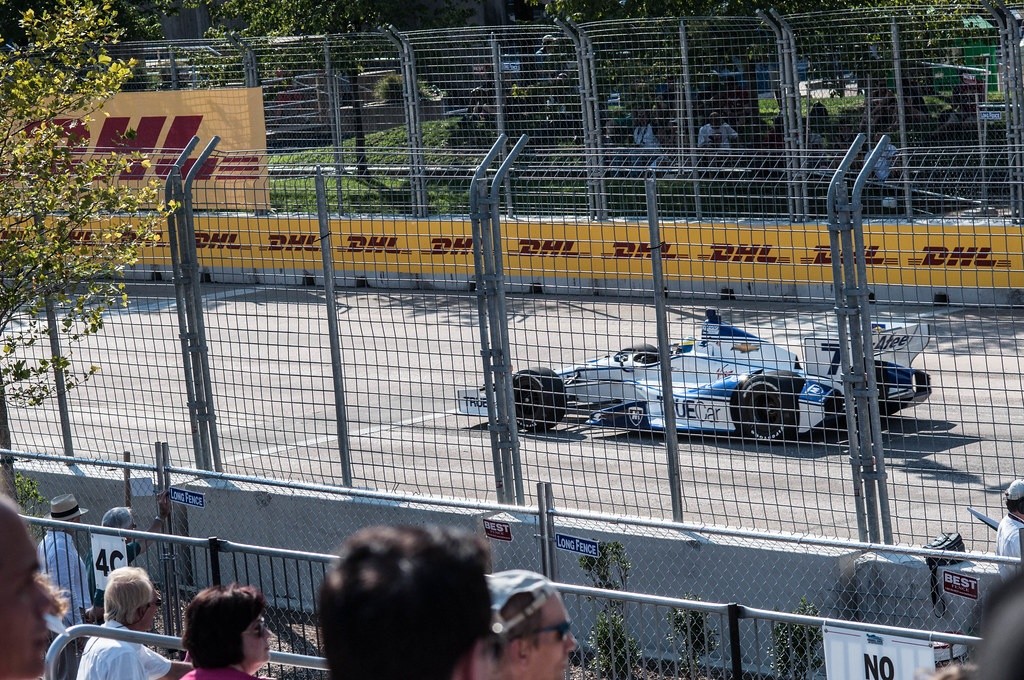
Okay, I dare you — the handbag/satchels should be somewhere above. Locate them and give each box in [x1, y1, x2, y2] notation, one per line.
[923, 532, 965, 618]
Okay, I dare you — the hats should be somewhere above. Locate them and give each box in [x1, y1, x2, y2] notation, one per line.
[485, 570, 557, 636]
[1003, 479, 1024, 500]
[43, 493, 90, 520]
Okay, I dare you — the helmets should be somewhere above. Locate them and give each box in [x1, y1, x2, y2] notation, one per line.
[676, 335, 697, 353]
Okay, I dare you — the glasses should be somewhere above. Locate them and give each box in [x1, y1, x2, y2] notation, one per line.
[149, 597, 162, 607]
[507, 617, 573, 643]
[128, 519, 137, 529]
[242, 616, 266, 638]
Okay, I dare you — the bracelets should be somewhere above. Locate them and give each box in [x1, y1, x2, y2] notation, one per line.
[155, 516, 164, 523]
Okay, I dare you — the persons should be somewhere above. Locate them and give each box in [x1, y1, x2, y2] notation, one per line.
[75, 566, 194, 680]
[178, 583, 271, 680]
[0, 493, 69, 680]
[454, 33, 986, 149]
[485, 570, 577, 680]
[924, 582, 1023, 680]
[35, 492, 92, 680]
[316, 525, 492, 680]
[994, 479, 1024, 606]
[87, 490, 171, 625]
[461, 36, 986, 216]
